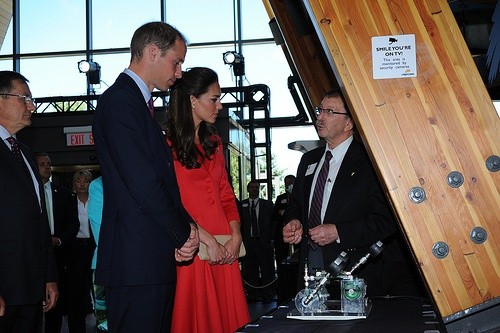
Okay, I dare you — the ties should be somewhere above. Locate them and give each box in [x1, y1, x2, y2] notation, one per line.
[308, 149, 333, 251]
[7, 135, 41, 214]
[44, 184, 51, 231]
[148, 96, 154, 118]
[251, 199, 259, 238]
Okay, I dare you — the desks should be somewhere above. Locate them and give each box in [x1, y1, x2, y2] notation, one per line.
[233, 296, 442, 333]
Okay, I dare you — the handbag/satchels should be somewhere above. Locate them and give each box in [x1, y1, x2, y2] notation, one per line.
[197, 233, 246, 260]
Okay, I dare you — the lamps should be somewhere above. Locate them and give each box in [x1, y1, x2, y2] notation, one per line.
[78, 60, 101, 84]
[223, 51, 245, 76]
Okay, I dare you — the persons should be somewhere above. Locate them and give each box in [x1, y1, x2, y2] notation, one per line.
[0, 69, 60, 333]
[152, 66, 251, 333]
[91, 20, 201, 333]
[281, 88, 418, 298]
[33, 152, 308, 333]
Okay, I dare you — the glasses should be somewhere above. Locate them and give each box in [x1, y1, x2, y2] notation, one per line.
[314, 106, 349, 117]
[0, 93, 36, 106]
